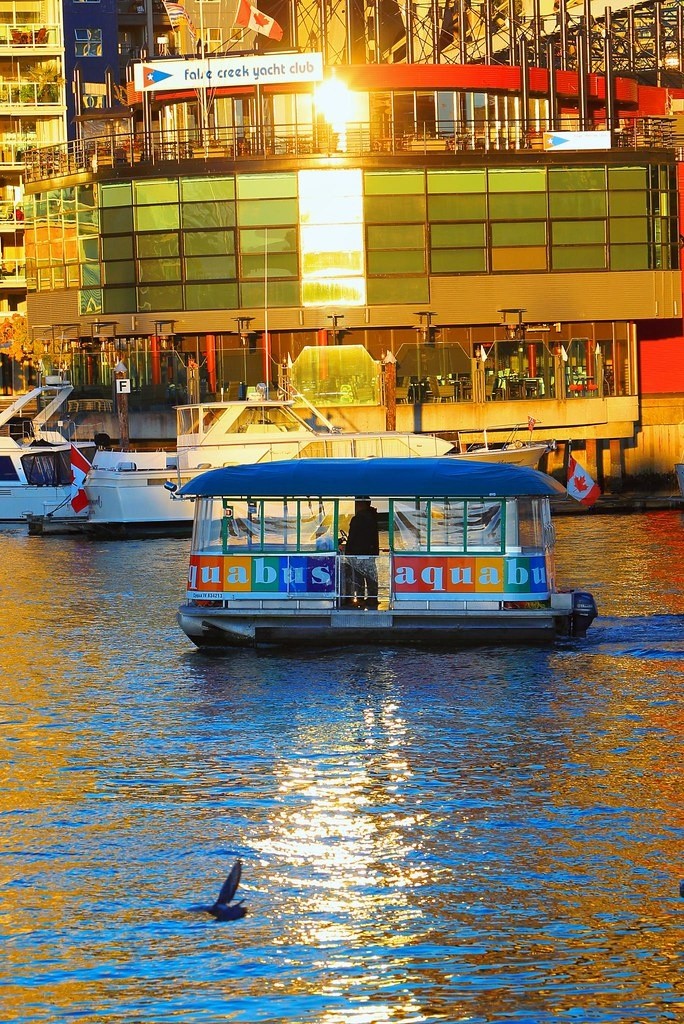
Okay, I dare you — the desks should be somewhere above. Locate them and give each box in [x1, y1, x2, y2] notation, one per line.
[64, 398, 114, 413]
[503, 377, 542, 400]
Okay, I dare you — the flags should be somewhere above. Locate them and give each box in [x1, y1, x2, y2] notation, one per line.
[161, 0, 196, 39]
[567, 452, 600, 508]
[560, 346, 569, 361]
[480, 345, 488, 363]
[70, 443, 90, 514]
[236, 0, 283, 41]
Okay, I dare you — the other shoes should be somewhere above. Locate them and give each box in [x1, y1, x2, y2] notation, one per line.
[354, 600, 366, 610]
[366, 598, 378, 610]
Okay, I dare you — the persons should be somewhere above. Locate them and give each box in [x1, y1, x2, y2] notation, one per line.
[344, 496, 380, 610]
[569, 366, 580, 398]
[188, 139, 194, 159]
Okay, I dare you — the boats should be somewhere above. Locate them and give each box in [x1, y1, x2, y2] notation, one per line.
[77, 384, 557, 542]
[0, 368, 95, 521]
[164, 460, 596, 645]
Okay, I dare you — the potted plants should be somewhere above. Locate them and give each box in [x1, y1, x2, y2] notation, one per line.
[19, 84, 36, 103]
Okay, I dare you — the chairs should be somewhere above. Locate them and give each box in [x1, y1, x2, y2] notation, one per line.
[428, 375, 456, 403]
[10, 28, 47, 46]
[389, 374, 411, 405]
[214, 380, 243, 403]
[482, 374, 496, 402]
